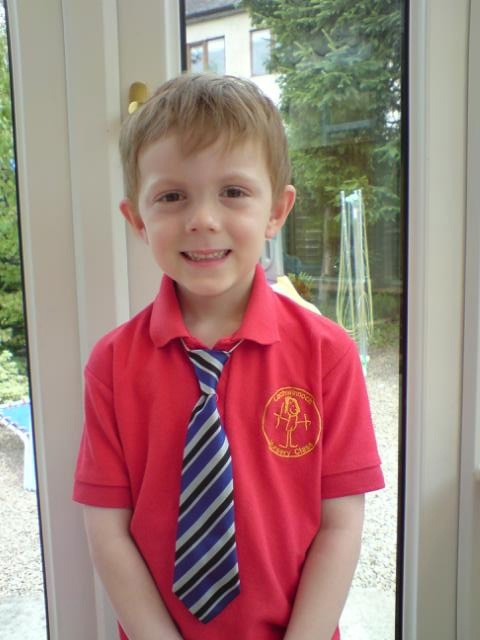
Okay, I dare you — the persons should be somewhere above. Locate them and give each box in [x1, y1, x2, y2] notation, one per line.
[72, 73, 385, 640]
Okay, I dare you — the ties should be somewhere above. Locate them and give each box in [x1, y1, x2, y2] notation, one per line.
[171, 340, 243, 626]
